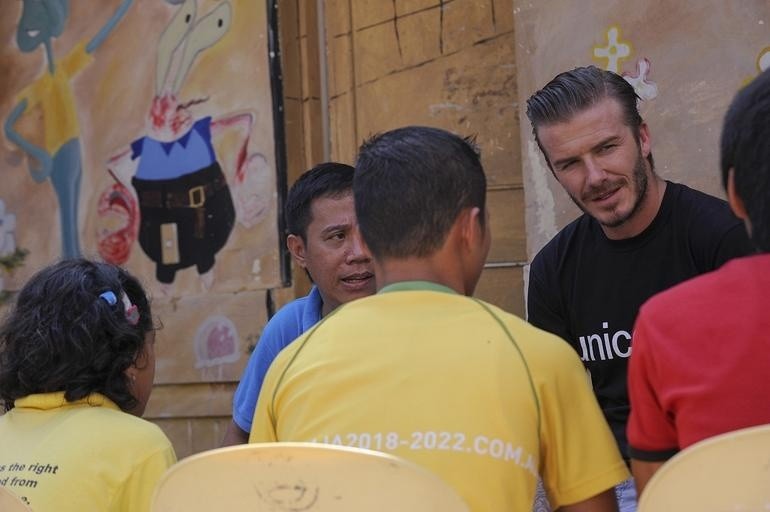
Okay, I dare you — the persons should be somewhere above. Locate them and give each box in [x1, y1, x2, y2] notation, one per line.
[624, 65, 769, 500]
[221, 159, 377, 448]
[521, 60, 750, 511]
[1, 259, 178, 512]
[241, 125, 632, 511]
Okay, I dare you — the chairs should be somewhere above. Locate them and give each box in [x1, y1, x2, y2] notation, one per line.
[149, 440, 480, 511]
[635, 424, 769, 510]
